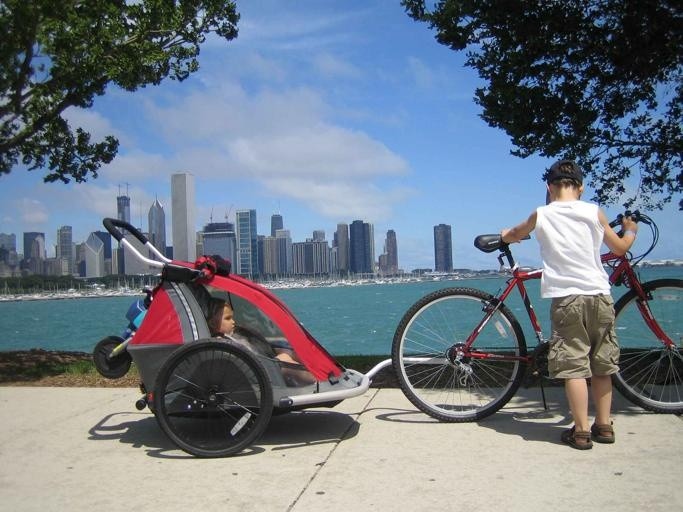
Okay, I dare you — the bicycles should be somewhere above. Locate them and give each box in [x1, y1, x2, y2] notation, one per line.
[389, 207, 683, 424]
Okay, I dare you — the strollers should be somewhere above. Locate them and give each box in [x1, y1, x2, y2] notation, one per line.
[90, 214, 372, 460]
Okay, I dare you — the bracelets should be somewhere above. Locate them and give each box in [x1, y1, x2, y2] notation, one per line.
[624, 231, 637, 237]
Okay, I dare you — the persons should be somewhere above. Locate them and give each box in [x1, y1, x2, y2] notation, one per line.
[499, 158, 640, 451]
[200, 296, 320, 386]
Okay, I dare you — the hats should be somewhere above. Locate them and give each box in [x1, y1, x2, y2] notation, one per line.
[547, 159, 583, 184]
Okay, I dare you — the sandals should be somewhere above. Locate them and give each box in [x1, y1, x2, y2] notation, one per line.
[561, 426, 593, 449]
[591, 423, 615, 443]
